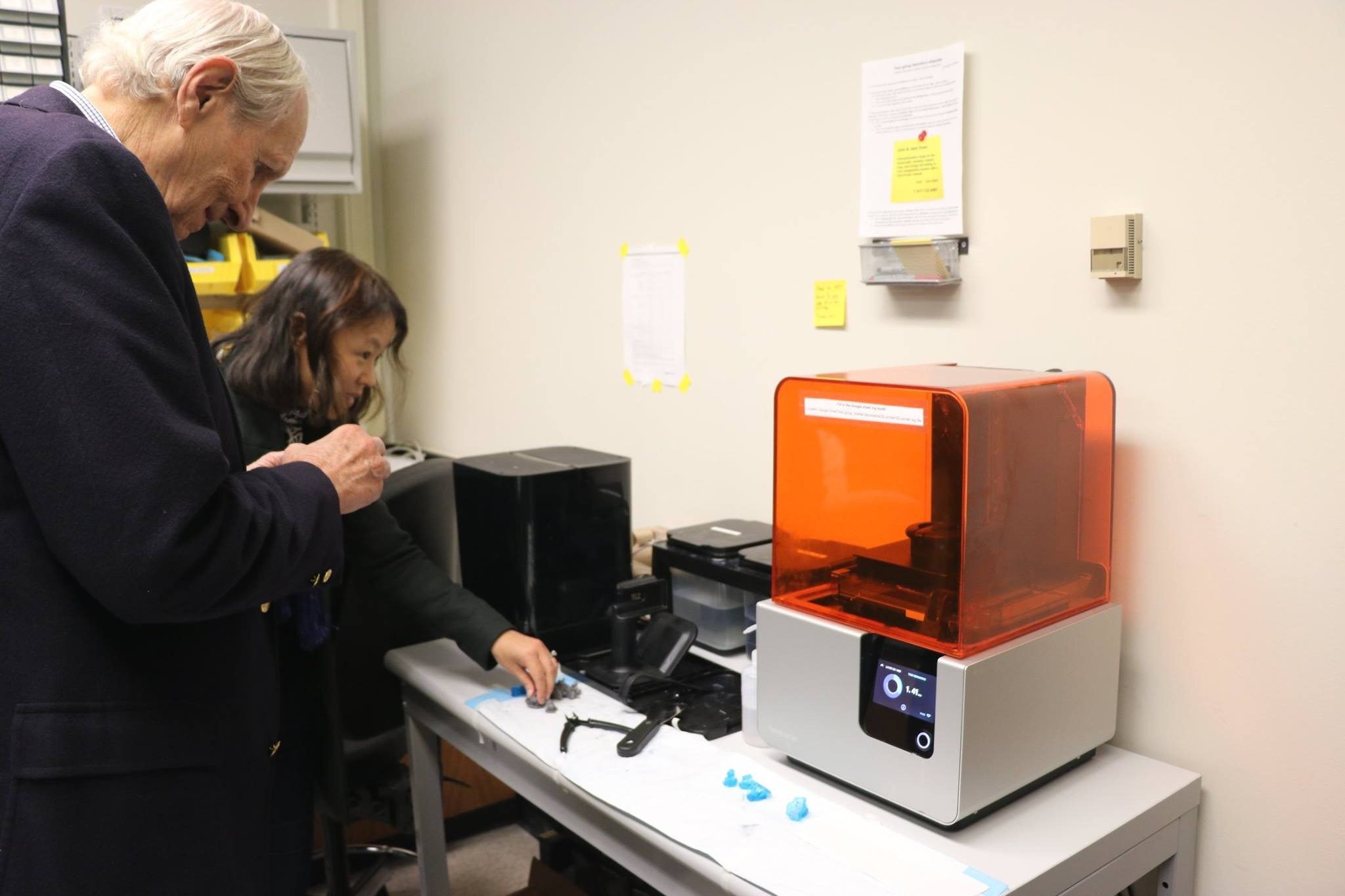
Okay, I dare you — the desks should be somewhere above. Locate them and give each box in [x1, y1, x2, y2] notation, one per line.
[384, 637, 1200, 896]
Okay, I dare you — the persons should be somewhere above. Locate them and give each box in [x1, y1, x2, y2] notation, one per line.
[0, 0, 389, 896]
[214, 244, 557, 896]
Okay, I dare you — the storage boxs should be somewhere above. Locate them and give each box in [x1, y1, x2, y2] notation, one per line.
[185, 196, 329, 344]
[527, 827, 669, 896]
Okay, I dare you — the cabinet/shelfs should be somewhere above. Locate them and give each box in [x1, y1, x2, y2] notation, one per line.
[258, 27, 362, 195]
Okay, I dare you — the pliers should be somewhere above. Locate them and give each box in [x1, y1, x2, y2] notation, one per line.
[560, 711, 633, 754]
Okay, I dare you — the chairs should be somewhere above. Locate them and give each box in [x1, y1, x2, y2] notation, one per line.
[307, 459, 466, 896]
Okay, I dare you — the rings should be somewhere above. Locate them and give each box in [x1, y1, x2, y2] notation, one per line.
[548, 650, 557, 659]
